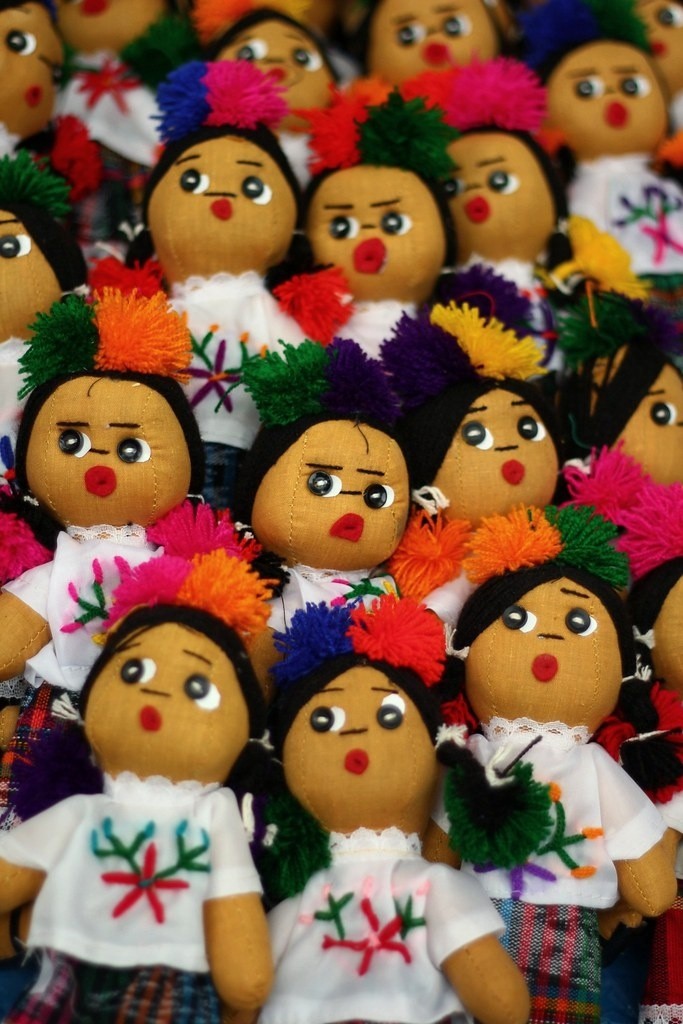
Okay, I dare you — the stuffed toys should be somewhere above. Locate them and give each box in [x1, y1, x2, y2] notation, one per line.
[422, 503, 678, 1024]
[0, 500, 272, 1024]
[0, 1, 683, 1024]
